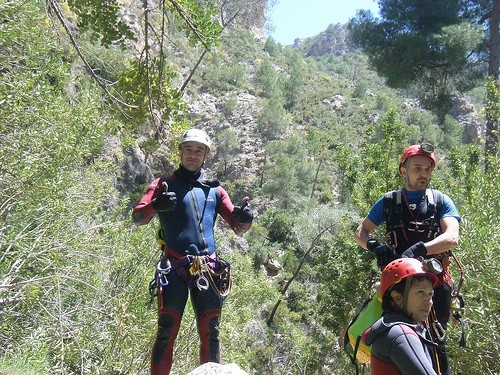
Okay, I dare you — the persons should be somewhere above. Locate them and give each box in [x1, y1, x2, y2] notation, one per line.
[133, 129, 253, 375]
[354, 144, 459, 375]
[371, 257, 438, 375]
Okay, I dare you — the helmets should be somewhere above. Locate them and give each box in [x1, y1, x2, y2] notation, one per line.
[381, 257, 443, 298]
[178, 128, 210, 155]
[399, 143, 437, 176]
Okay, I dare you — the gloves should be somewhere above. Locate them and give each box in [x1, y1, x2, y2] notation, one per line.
[153, 182, 177, 212]
[402, 241, 427, 258]
[232, 196, 254, 224]
[369, 244, 394, 259]
